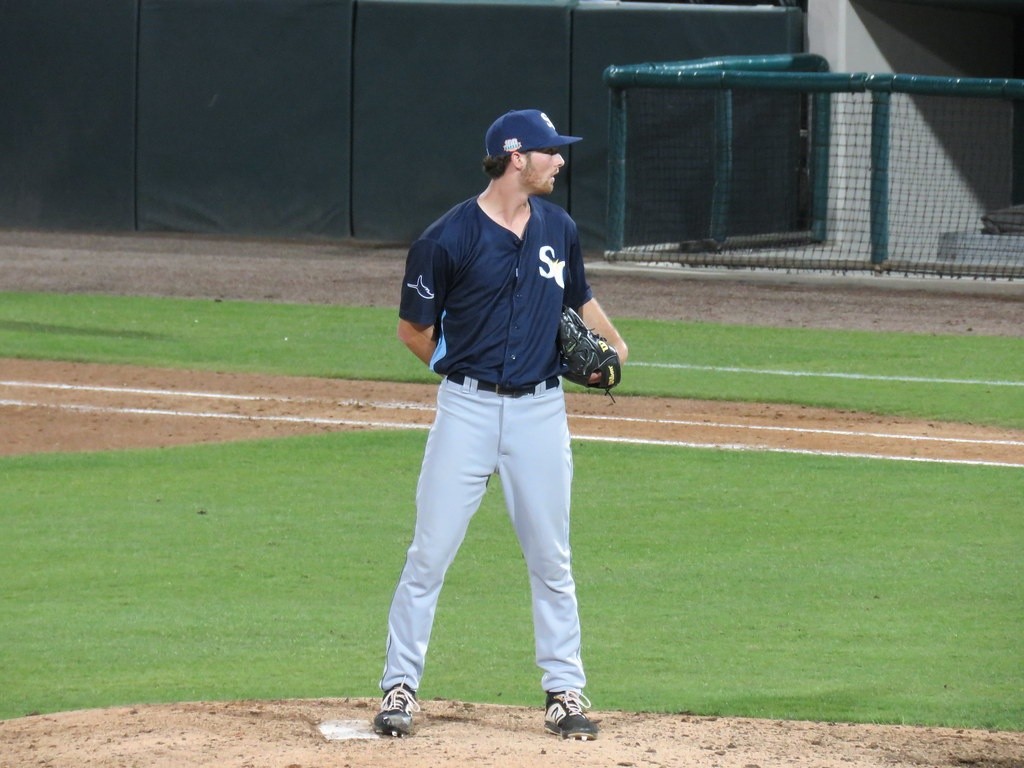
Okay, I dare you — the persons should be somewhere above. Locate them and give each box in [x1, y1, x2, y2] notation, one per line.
[370, 109, 628, 744]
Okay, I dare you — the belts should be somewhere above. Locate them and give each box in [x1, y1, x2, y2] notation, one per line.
[446, 372, 559, 399]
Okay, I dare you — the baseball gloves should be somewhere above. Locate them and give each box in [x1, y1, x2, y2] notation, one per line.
[554, 304, 623, 407]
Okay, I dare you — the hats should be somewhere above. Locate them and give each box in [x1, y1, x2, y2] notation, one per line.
[485, 109, 584, 153]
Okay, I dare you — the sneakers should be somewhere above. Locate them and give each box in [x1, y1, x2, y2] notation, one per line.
[543, 692, 599, 741]
[375, 687, 415, 738]
